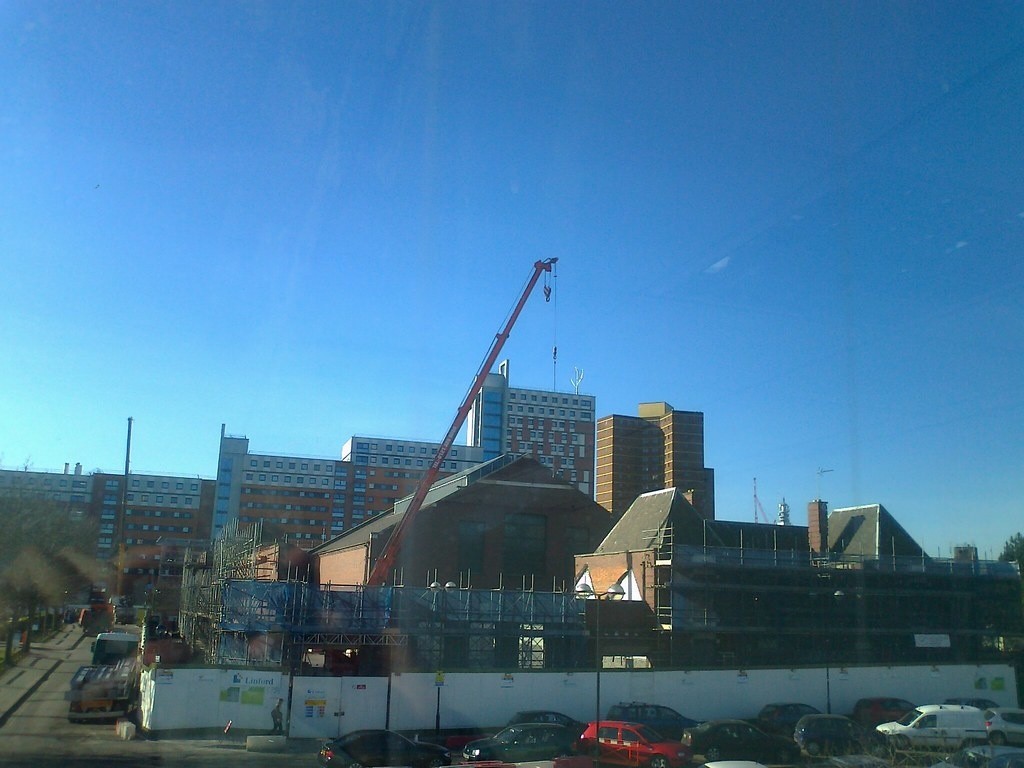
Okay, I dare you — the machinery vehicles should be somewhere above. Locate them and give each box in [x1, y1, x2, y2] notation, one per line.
[77, 585, 113, 636]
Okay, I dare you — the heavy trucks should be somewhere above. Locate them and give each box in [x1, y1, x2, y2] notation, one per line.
[64, 633, 139, 724]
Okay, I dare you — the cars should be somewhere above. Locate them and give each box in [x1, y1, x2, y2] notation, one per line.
[580, 718, 692, 768]
[464, 722, 578, 762]
[791, 714, 892, 758]
[983, 707, 1024, 746]
[319, 728, 451, 768]
[509, 696, 1000, 729]
[680, 718, 800, 763]
[929, 745, 1024, 767]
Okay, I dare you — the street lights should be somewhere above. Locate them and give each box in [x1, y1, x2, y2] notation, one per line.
[430, 581, 457, 737]
[575, 583, 625, 768]
[808, 591, 845, 714]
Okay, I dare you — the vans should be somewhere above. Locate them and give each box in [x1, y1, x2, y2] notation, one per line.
[877, 705, 990, 750]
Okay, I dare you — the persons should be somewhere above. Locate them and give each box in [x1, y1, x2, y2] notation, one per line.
[270, 704, 282, 735]
